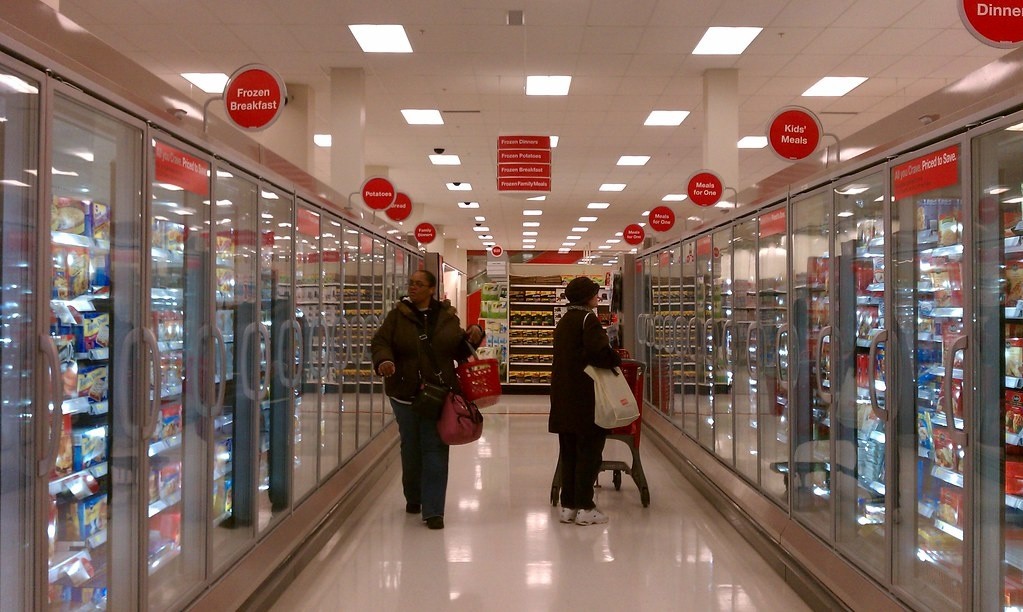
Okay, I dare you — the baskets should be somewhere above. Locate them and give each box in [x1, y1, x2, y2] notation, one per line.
[455, 342, 502, 409]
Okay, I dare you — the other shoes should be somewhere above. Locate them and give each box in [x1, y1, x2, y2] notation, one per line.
[426, 510, 444, 529]
[406, 497, 419, 512]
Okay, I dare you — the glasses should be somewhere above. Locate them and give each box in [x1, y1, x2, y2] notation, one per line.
[405, 279, 433, 288]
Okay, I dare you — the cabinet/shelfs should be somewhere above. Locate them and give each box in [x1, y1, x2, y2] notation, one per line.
[506, 262, 611, 385]
[282, 284, 384, 385]
[652, 285, 732, 387]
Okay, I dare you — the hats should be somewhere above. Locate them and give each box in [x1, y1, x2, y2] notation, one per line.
[565, 276, 599, 305]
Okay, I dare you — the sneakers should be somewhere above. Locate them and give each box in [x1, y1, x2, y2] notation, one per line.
[575, 509, 608, 526]
[559, 508, 576, 523]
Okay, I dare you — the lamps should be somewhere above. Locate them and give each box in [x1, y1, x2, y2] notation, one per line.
[173, 108, 187, 120]
[920, 115, 934, 124]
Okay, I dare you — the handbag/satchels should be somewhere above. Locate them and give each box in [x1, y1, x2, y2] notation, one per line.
[437, 392, 484, 446]
[411, 324, 451, 421]
[584, 362, 639, 429]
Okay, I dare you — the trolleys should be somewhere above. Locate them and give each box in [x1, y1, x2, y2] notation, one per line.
[549, 348, 651, 509]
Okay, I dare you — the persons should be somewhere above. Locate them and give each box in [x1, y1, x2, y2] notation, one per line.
[370, 270, 485, 529]
[547, 276, 622, 525]
